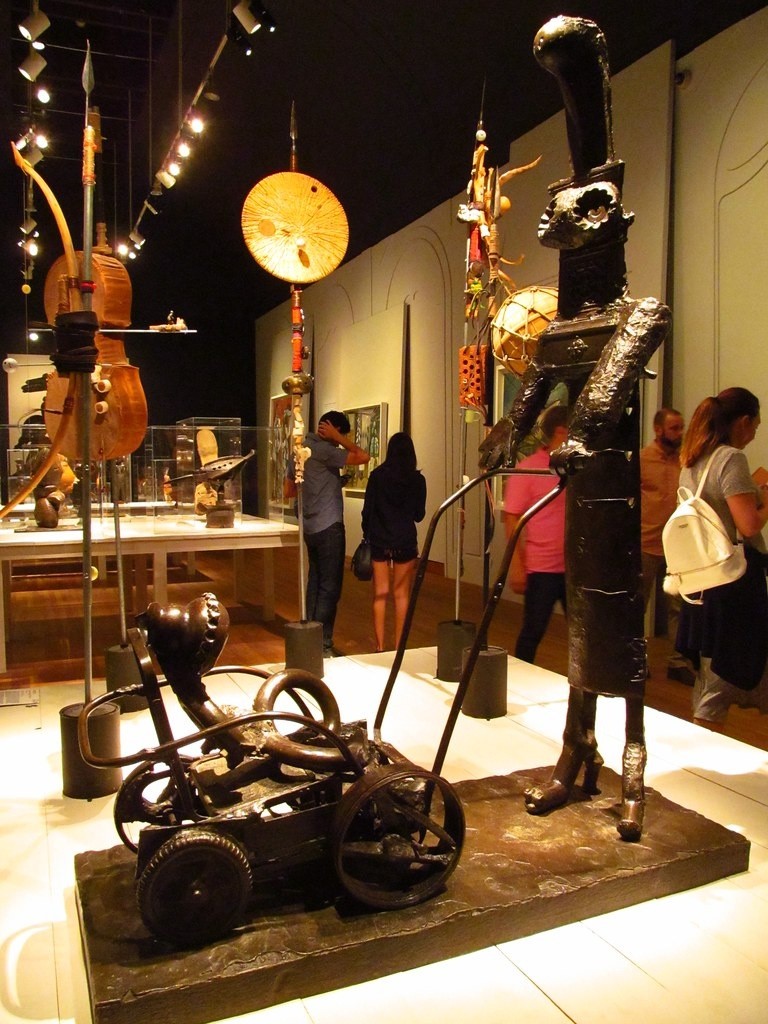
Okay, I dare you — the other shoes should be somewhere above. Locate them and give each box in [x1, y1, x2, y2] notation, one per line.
[667, 667, 697, 685]
[323, 648, 345, 658]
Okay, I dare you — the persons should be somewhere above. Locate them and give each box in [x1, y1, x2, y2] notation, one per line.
[640, 408, 685, 628]
[506, 406, 567, 664]
[284, 411, 371, 659]
[8, 414, 55, 492]
[675, 386, 768, 733]
[360, 432, 426, 652]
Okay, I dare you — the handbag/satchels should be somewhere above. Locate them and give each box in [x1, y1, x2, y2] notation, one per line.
[351, 540, 371, 581]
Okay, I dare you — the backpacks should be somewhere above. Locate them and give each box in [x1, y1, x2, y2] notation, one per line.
[661, 445, 747, 604]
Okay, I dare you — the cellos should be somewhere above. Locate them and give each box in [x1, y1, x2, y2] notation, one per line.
[42, 111, 150, 525]
